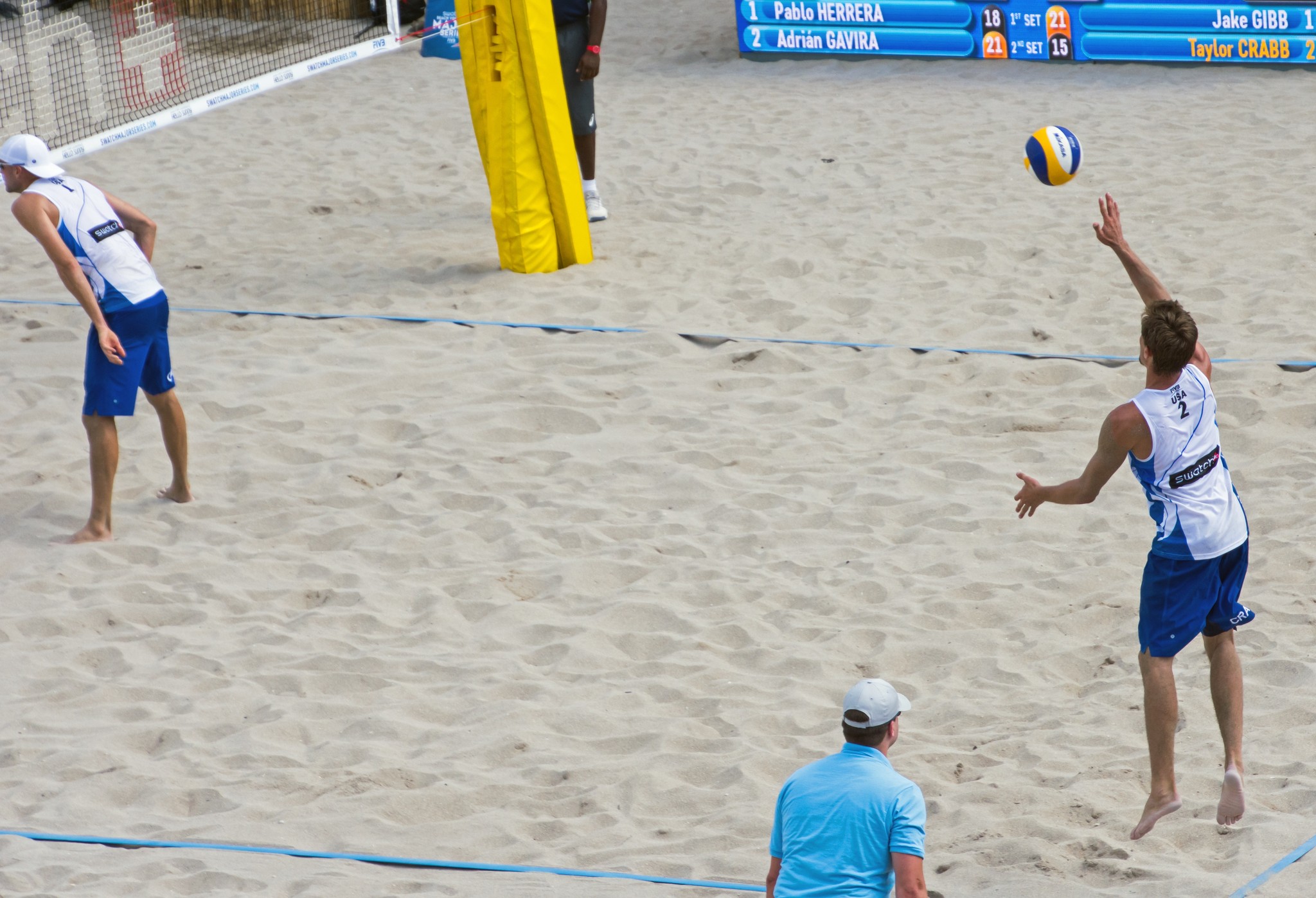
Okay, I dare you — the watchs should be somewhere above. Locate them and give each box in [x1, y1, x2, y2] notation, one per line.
[586, 45, 600, 54]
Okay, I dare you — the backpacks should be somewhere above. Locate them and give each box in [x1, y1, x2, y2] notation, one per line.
[354, 0, 426, 38]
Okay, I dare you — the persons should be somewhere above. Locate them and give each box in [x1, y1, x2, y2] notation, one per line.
[551, 0, 608, 221]
[0, 136, 192, 544]
[767, 678, 945, 898]
[1015, 193, 1257, 839]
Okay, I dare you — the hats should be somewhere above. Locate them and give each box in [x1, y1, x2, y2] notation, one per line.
[842, 678, 912, 729]
[0, 133, 66, 179]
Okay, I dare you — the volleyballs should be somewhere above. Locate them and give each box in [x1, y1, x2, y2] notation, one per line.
[1023, 124, 1083, 187]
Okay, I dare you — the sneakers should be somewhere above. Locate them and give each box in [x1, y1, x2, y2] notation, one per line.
[584, 190, 608, 222]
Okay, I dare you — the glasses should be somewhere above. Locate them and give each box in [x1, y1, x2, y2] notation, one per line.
[0, 162, 25, 172]
